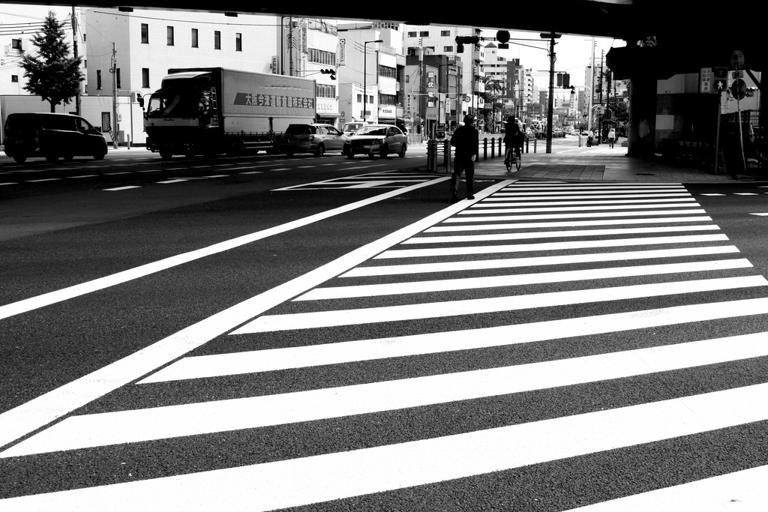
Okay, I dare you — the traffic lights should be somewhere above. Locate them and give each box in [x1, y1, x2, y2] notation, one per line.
[570, 85, 576, 94]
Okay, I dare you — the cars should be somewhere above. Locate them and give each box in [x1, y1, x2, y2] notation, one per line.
[342, 124, 407, 160]
[538, 126, 606, 147]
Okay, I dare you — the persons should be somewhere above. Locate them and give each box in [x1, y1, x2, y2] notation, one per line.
[449, 113, 480, 199]
[500, 115, 526, 168]
[607, 128, 616, 149]
[586, 128, 595, 148]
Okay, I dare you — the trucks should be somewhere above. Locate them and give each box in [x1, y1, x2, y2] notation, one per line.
[138, 66, 317, 160]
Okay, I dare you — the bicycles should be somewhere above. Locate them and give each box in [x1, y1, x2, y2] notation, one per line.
[502, 145, 522, 174]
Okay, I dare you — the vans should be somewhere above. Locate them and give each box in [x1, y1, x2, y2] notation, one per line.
[340, 121, 368, 137]
[281, 122, 350, 157]
[2, 112, 107, 162]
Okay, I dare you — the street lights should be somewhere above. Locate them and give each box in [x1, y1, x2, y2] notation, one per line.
[363, 39, 383, 122]
[586, 65, 603, 144]
